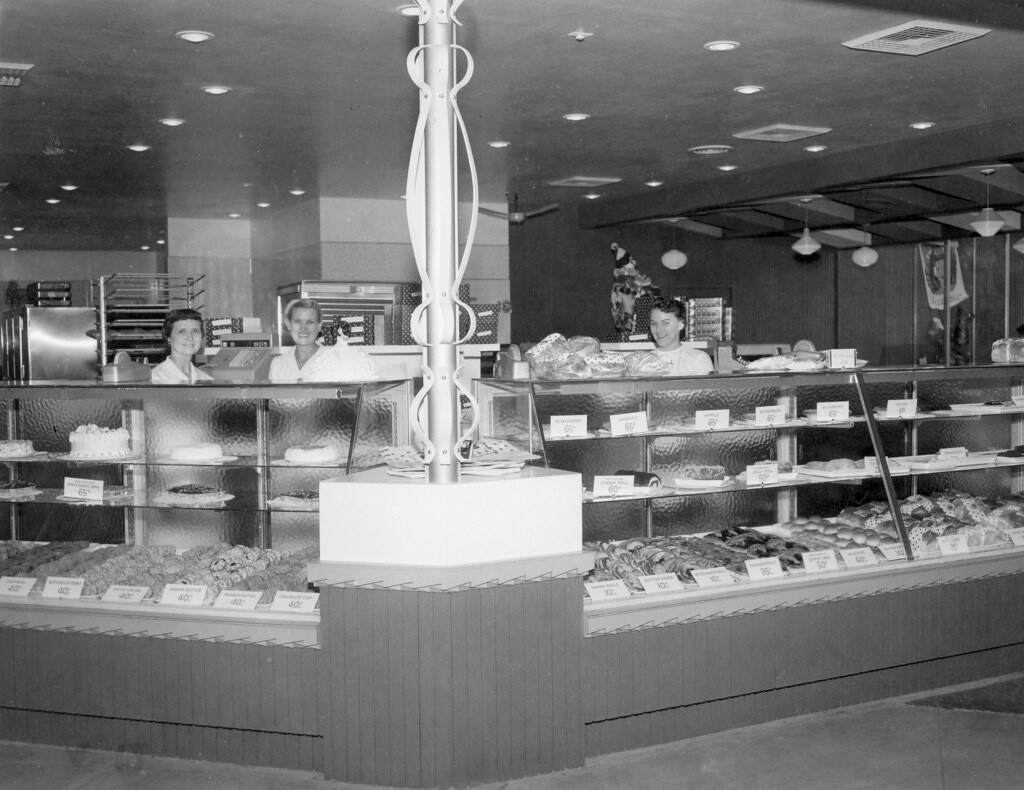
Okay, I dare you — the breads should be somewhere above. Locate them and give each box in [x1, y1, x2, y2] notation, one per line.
[990, 337, 1024, 362]
[582, 485, 1024, 593]
[528, 332, 830, 381]
[613, 459, 877, 489]
[0, 538, 320, 604]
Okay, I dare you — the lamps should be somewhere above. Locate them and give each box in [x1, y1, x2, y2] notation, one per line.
[661, 217, 688, 270]
[970, 169, 1005, 238]
[852, 223, 878, 267]
[792, 199, 822, 255]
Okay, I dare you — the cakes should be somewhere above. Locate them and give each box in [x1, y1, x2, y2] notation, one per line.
[0, 422, 340, 507]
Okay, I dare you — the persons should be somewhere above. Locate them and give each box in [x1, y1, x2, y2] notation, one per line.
[139, 310, 230, 544]
[930, 247, 957, 295]
[268, 297, 355, 440]
[649, 300, 716, 376]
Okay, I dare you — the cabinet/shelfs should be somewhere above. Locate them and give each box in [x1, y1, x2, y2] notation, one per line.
[0, 378, 414, 738]
[469, 364, 1023, 723]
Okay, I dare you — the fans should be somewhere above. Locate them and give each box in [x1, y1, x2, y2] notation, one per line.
[479, 190, 561, 222]
